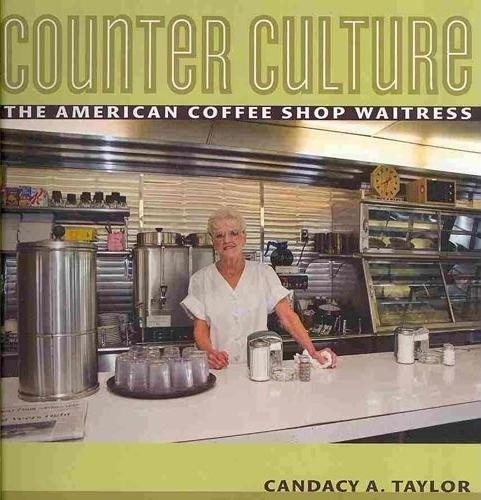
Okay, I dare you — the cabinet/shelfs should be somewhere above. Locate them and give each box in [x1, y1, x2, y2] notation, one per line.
[0, 203, 134, 258]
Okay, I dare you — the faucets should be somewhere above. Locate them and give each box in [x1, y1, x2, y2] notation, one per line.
[157, 285, 167, 309]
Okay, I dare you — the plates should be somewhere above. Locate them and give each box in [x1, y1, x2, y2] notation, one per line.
[96, 313, 121, 347]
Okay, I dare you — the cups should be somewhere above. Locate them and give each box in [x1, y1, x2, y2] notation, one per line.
[115, 344, 209, 393]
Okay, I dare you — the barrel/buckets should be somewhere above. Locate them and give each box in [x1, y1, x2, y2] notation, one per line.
[131, 245, 190, 329]
[189, 246, 214, 276]
[15, 235, 100, 403]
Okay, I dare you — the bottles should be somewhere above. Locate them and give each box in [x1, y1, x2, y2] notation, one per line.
[394, 327, 429, 363]
[249, 338, 271, 382]
[300, 357, 310, 381]
[443, 344, 455, 366]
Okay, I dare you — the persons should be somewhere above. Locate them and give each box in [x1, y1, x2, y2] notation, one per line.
[180, 206, 337, 369]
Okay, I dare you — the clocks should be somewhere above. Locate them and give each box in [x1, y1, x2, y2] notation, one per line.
[371, 162, 403, 201]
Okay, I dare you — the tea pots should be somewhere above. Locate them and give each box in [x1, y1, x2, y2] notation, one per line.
[265, 241, 294, 266]
